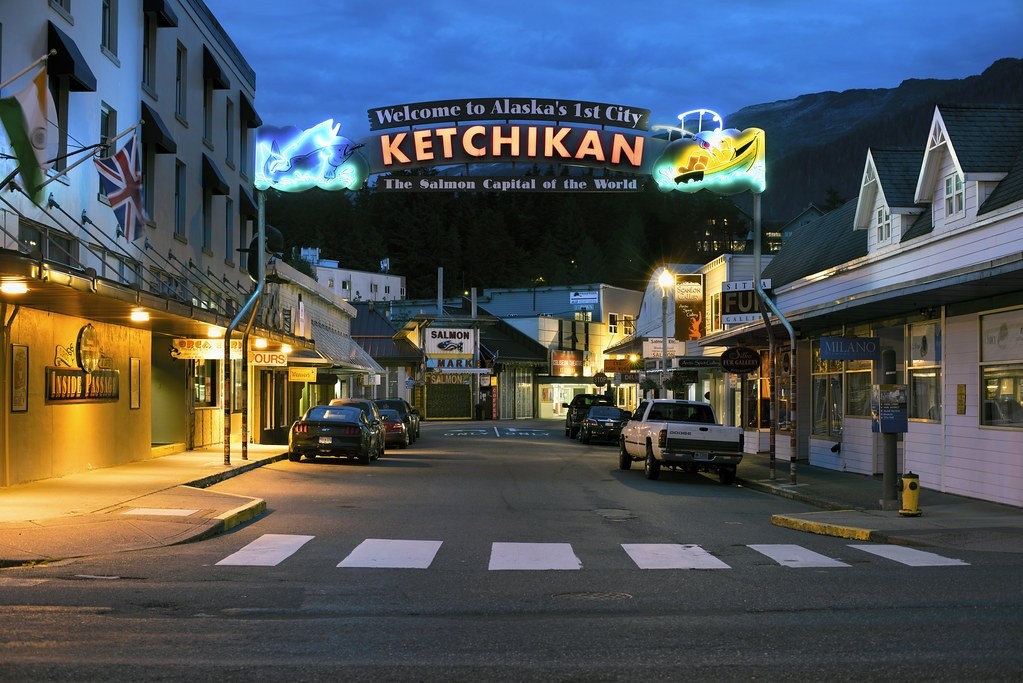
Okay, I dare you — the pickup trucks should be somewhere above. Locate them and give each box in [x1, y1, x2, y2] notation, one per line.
[562, 394, 614, 440]
[616, 398, 746, 485]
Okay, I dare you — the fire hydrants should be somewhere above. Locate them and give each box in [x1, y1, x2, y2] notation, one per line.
[895, 471, 924, 517]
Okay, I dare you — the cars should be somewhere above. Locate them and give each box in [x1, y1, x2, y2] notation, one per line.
[577, 406, 630, 442]
[380, 410, 410, 448]
[320, 396, 387, 459]
[371, 397, 421, 442]
[286, 405, 380, 464]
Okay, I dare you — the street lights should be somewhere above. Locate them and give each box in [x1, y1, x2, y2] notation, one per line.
[657, 269, 671, 399]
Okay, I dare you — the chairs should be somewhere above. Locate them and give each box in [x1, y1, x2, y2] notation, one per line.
[690, 414, 703, 422]
[650, 410, 664, 420]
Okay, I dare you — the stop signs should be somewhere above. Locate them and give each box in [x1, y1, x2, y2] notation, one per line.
[594, 372, 608, 387]
